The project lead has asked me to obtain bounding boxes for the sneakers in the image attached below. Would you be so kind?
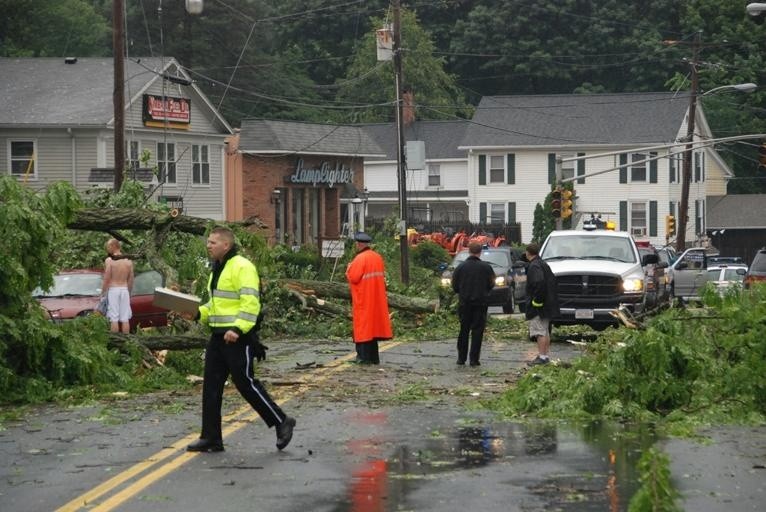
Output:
[188,437,224,452]
[528,357,549,366]
[276,417,296,449]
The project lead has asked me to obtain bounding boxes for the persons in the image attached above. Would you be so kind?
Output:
[452,243,496,364]
[187,226,296,453]
[525,243,559,367]
[101,237,134,336]
[345,233,392,364]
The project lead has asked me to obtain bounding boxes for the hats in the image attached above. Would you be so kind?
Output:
[355,235,371,243]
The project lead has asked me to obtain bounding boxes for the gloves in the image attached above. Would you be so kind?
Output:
[255,341,268,362]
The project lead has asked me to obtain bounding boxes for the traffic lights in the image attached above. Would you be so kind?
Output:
[551,187,563,219]
[665,215,675,238]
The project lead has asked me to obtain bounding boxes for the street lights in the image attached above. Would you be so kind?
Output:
[746,2,766,18]
[675,82,757,257]
[109,0,205,196]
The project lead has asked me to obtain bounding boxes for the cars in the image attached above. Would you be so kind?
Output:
[29,268,170,333]
[440,245,529,313]
[537,220,766,330]
